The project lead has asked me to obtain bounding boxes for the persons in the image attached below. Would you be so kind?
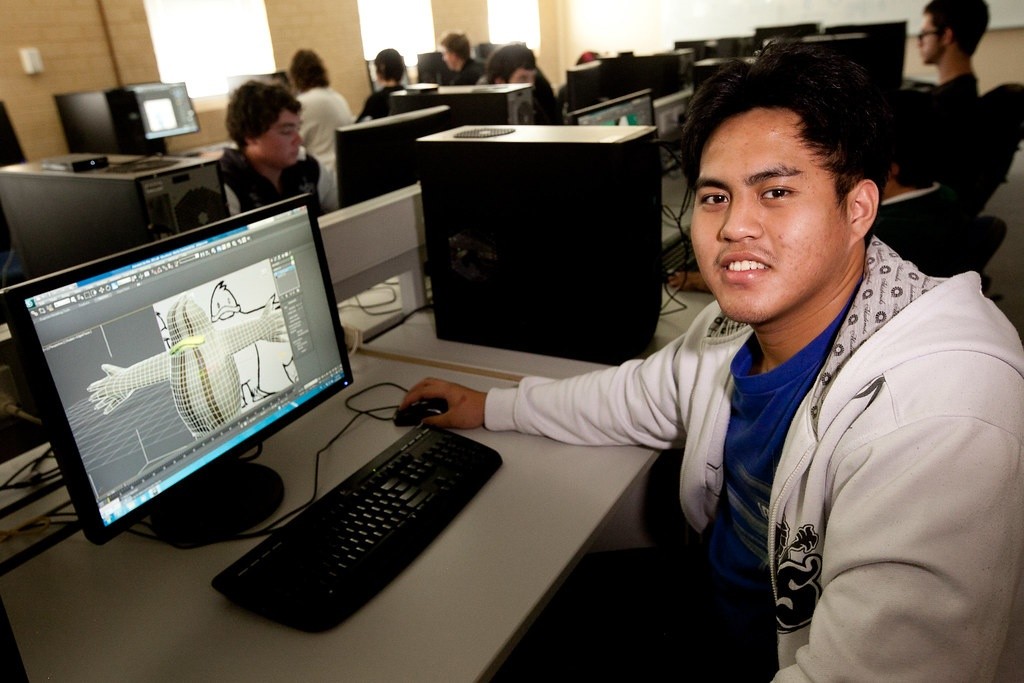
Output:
[915,0,991,138]
[289,49,357,208]
[399,34,1024,682]
[355,49,407,127]
[424,34,609,120]
[217,80,339,218]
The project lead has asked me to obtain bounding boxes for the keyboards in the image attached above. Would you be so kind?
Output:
[660,222,700,285]
[213,423,503,634]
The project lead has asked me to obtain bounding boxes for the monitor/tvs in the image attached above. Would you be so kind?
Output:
[366,60,411,94]
[565,89,655,127]
[244,73,291,93]
[565,60,603,111]
[334,106,449,209]
[125,82,200,140]
[0,190,355,551]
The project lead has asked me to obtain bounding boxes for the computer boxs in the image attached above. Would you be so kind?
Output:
[0,20,907,367]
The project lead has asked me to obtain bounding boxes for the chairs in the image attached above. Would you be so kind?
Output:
[916,83,1024,283]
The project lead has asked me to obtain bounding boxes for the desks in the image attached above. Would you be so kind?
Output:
[0,169,717,683]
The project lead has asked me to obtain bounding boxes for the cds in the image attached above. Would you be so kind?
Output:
[455,127,516,139]
[104,159,179,173]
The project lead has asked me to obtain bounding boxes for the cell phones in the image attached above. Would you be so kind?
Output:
[43,153,109,172]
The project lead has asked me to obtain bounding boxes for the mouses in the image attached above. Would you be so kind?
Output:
[392,398,448,428]
